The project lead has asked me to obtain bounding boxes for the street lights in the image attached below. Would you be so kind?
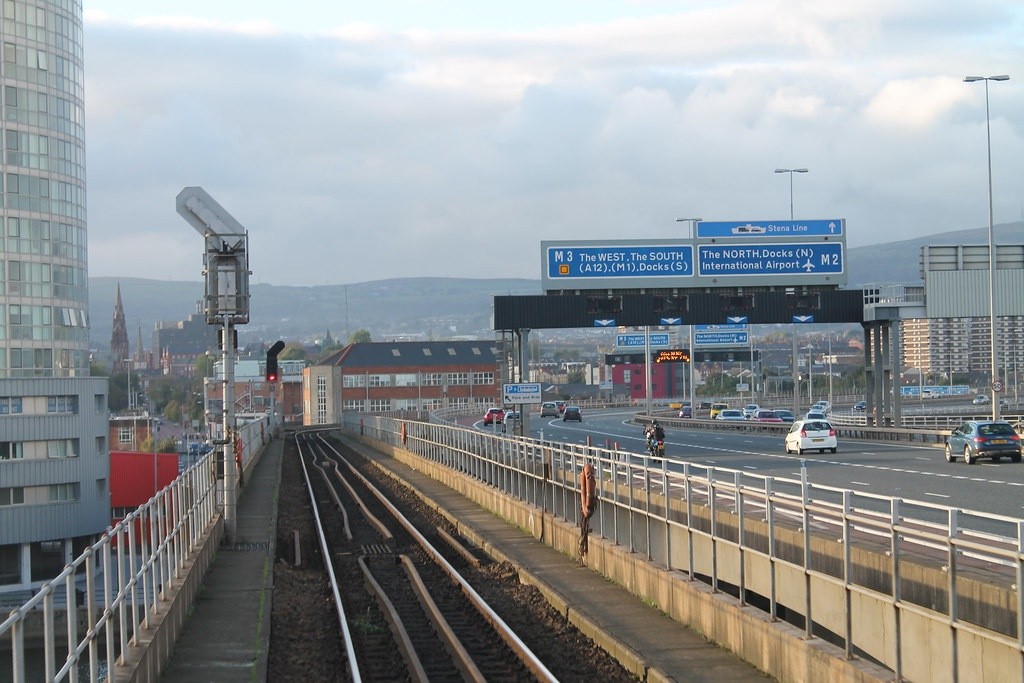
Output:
[945,338,952,396]
[827,330,835,406]
[774,167,809,220]
[962,75,1012,422]
[675,217,703,238]
[124,358,134,440]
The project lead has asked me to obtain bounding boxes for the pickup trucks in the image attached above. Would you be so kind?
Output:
[922,390,941,399]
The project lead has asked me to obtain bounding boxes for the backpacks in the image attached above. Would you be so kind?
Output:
[652,427,665,441]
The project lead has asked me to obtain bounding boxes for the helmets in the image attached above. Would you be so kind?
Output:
[654,424,659,428]
[651,418,657,423]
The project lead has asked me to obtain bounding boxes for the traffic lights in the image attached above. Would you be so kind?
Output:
[266,340,286,384]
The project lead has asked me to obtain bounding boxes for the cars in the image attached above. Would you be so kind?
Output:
[553,400,570,414]
[563,405,583,422]
[943,421,1022,465]
[153,417,214,476]
[784,419,838,455]
[1011,422,1024,435]
[500,410,521,433]
[972,395,990,405]
[539,402,560,419]
[853,401,867,412]
[668,400,831,432]
[483,407,505,427]
[1000,400,1008,410]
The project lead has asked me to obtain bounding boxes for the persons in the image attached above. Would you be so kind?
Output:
[643,418,665,456]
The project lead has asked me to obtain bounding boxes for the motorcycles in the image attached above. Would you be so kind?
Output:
[640,425,665,463]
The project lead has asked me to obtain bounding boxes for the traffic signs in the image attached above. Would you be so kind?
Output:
[502,383,542,405]
[697,218,844,238]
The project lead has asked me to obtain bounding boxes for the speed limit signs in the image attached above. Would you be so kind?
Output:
[991,381,1003,392]
